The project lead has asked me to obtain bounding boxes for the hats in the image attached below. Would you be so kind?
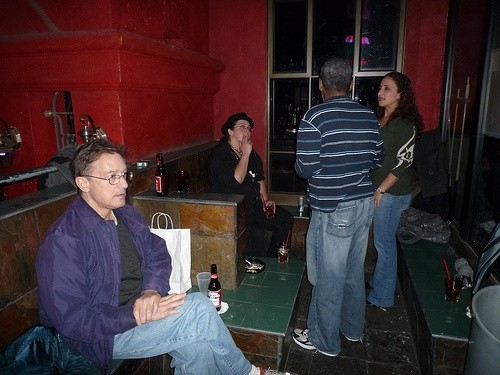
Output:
[221,112,254,137]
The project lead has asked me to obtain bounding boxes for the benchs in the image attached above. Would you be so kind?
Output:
[275,204,313,260]
[186,254,306,375]
[155,139,220,192]
[397,236,484,375]
[0,183,164,375]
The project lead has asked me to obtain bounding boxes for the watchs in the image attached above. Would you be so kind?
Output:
[377,188,385,194]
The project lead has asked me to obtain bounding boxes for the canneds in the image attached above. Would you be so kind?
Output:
[298,205,304,213]
[298,196,304,205]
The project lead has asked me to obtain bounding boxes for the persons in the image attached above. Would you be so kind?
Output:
[35,138,297,375]
[203,113,294,257]
[366,71,425,307]
[293,55,384,357]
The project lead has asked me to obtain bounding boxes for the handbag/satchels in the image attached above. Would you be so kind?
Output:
[148,212,192,295]
[396,206,451,244]
[0,326,102,375]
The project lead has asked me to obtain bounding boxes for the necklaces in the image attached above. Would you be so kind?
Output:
[231,145,240,154]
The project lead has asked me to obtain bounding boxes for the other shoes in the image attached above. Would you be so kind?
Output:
[260,365,297,375]
[366,300,372,305]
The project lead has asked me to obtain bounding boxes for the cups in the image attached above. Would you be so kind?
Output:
[446,274,461,299]
[196,271,210,296]
[278,241,289,264]
[175,169,189,194]
[264,202,274,218]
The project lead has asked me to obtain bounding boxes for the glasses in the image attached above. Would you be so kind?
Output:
[234,125,250,130]
[81,172,133,185]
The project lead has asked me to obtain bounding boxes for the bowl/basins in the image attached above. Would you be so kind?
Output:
[245,259,266,273]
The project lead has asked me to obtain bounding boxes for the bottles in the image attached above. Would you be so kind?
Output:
[206,263,222,311]
[155,153,169,197]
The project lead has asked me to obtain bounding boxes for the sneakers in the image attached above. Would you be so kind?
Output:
[292,328,341,356]
[345,335,359,341]
